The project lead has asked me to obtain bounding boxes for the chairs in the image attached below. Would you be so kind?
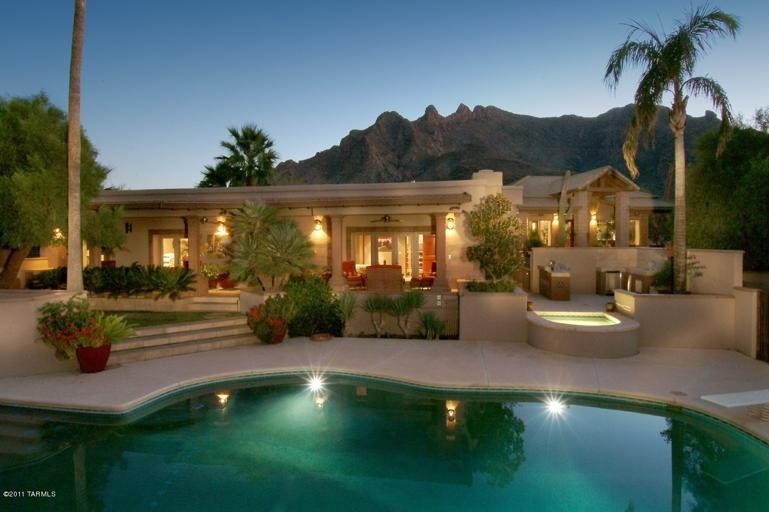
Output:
[343,260,438,290]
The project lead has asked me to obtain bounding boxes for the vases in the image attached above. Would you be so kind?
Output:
[77,343,111,373]
[655,286,692,294]
[259,321,293,344]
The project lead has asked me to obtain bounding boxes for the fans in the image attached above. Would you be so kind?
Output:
[369,215,406,225]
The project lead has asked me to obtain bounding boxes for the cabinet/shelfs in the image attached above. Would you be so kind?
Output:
[539,265,570,301]
[595,266,657,297]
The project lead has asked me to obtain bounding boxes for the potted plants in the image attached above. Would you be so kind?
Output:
[458,194,529,344]
[290,276,344,342]
[203,263,239,289]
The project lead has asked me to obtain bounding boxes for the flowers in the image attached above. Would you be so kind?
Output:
[647,240,676,285]
[32,296,141,358]
[245,294,296,331]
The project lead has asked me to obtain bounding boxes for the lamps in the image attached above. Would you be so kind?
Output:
[313,215,324,230]
[216,219,225,234]
[447,213,456,231]
